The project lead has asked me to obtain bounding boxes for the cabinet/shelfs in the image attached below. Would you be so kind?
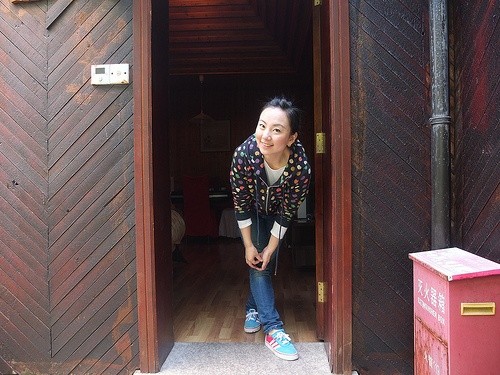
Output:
[287,220,315,272]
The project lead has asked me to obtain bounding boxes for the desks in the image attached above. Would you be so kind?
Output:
[173,194,184,199]
[209,188,230,197]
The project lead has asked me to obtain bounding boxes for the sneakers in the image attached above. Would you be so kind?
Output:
[244,309,261,333]
[265,329,298,361]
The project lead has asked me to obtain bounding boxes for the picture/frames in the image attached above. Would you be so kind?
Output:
[199,118,232,152]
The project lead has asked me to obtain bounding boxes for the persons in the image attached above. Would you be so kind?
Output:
[229,97,311,361]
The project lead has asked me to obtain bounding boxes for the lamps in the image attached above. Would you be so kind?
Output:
[188,75,216,125]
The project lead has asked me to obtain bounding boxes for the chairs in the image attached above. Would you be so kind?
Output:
[181,176,219,241]
